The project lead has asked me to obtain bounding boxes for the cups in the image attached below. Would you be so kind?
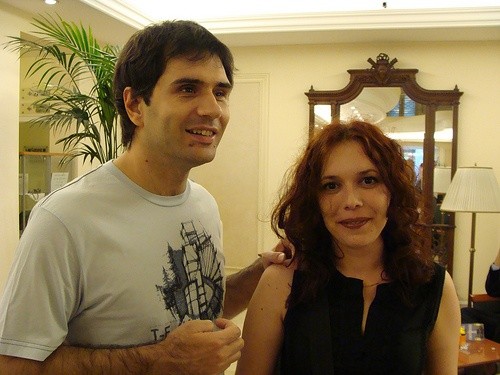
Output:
[460,323,485,358]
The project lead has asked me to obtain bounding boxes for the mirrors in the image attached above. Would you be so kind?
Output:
[303,57,464,282]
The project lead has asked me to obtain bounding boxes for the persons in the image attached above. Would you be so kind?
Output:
[235,120,461,375]
[469,249,500,344]
[0,19,296,375]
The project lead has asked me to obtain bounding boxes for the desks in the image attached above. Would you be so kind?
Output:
[457,328,500,375]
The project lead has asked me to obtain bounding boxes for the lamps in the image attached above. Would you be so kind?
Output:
[439,163,500,309]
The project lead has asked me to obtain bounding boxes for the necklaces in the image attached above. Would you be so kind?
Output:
[363,278,385,288]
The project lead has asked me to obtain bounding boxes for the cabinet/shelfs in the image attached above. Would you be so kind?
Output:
[18,150,76,238]
